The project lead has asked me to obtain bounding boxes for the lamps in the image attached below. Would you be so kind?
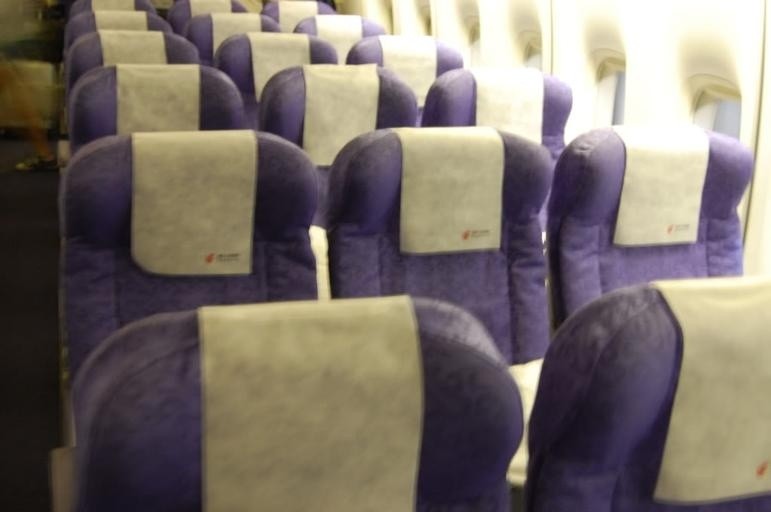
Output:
[65,28,201,100]
[56,130,316,388]
[419,67,575,234]
[61,293,525,512]
[182,12,282,67]
[258,64,418,234]
[293,14,388,65]
[546,120,755,328]
[214,29,339,130]
[526,274,771,512]
[324,125,554,511]
[260,1,339,33]
[344,33,464,126]
[67,0,159,21]
[63,9,176,53]
[65,62,246,159]
[166,0,251,36]
